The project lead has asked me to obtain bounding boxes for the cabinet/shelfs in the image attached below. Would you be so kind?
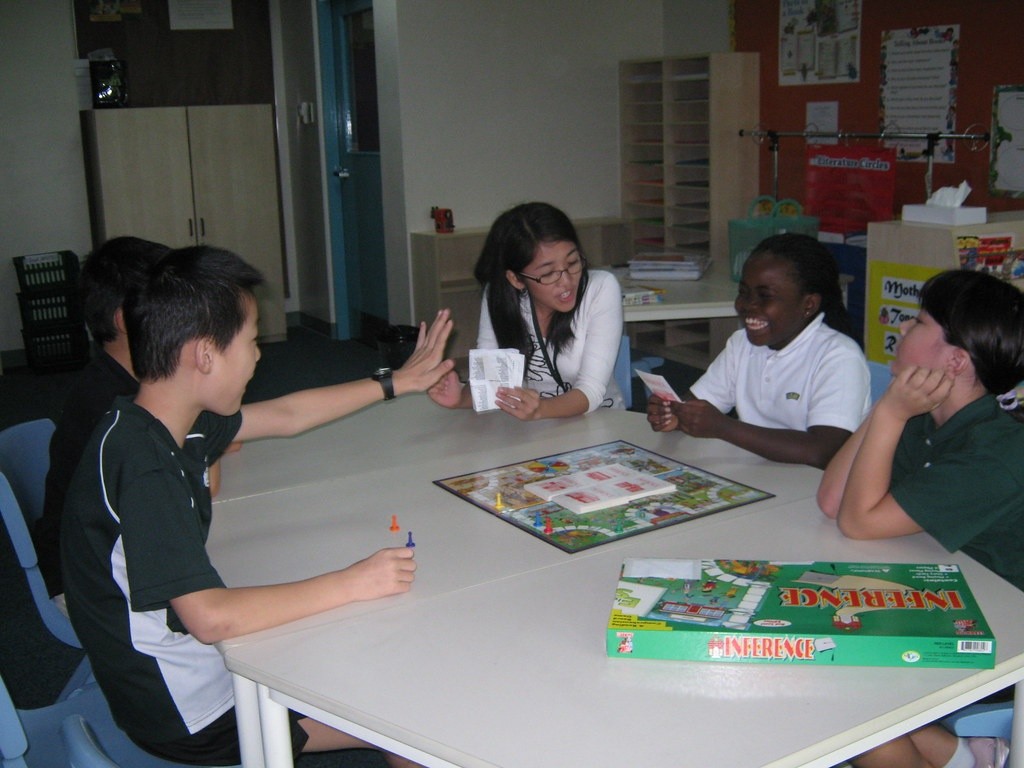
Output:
[618,51,761,370]
[80,103,287,344]
[410,217,635,363]
[865,210,1024,368]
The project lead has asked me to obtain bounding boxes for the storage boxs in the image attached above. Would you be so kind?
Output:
[13,250,90,372]
[605,556,996,669]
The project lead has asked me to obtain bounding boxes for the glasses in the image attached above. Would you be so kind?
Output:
[518,256,587,285]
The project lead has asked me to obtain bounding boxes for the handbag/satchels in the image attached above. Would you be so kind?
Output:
[727,196,820,282]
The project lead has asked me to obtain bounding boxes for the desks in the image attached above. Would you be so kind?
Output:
[208,383,1024,768]
[595,258,854,322]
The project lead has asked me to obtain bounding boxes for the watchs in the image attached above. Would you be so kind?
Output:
[372,367,396,400]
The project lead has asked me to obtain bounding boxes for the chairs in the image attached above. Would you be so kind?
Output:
[0,675,202,768]
[0,417,97,707]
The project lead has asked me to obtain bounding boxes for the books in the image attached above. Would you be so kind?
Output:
[620,251,713,305]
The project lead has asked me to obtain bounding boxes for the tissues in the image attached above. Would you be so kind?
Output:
[901,179,987,225]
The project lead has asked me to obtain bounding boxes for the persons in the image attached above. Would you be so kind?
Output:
[816,269,1024,768]
[59,246,455,768]
[425,202,626,421]
[39,236,174,620]
[646,233,873,470]
